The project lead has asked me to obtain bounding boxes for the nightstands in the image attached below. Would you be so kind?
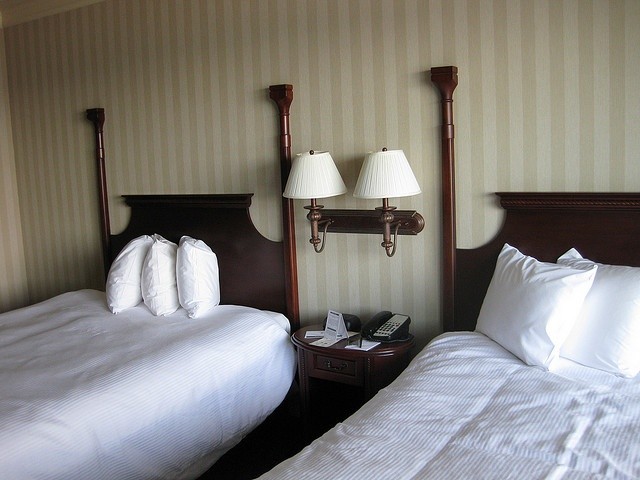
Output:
[291,325,416,400]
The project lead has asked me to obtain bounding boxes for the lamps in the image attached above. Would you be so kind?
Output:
[282,150,347,254]
[353,146,421,257]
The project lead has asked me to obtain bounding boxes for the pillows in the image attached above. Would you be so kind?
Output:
[141,239,179,316]
[554,245,640,379]
[105,235,153,313]
[475,242,599,371]
[176,235,220,319]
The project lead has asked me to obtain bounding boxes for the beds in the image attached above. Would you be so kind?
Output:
[257,67,640,479]
[0,83,298,479]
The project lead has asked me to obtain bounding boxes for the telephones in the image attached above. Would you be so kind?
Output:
[361,311,411,342]
